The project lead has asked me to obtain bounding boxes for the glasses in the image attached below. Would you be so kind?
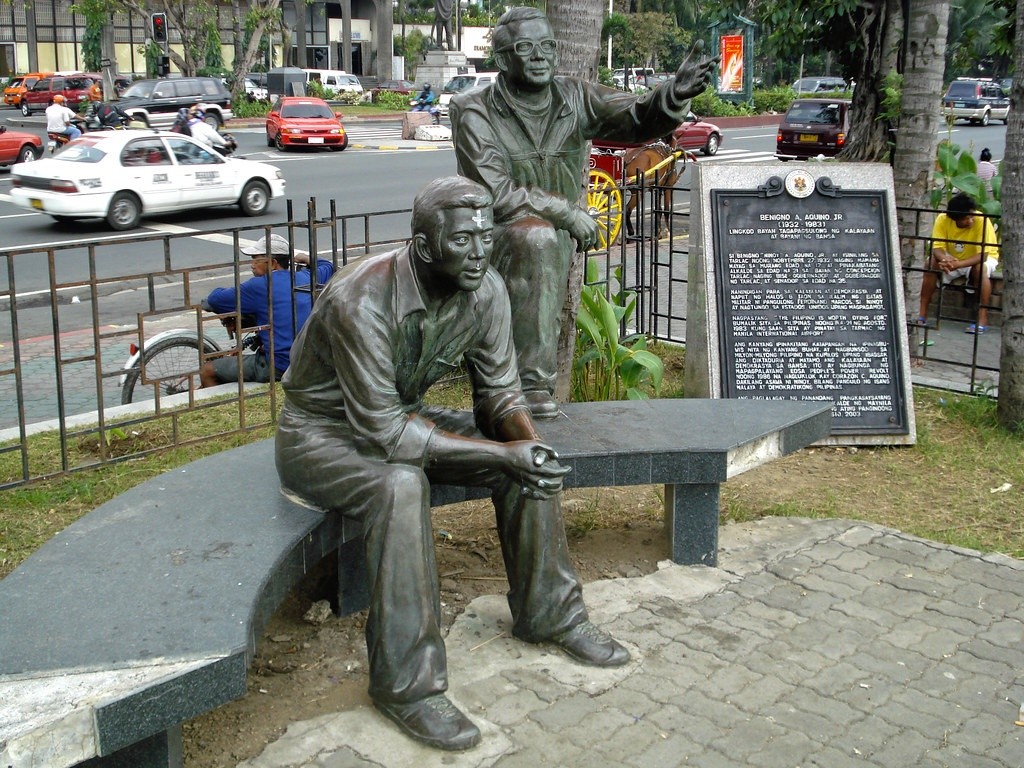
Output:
[252,259,269,265]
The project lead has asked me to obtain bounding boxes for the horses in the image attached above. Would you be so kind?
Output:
[623,132,684,237]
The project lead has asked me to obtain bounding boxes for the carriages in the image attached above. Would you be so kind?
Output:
[587,132,704,251]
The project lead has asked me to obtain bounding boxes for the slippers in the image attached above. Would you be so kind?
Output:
[964,324,989,335]
[912,318,931,326]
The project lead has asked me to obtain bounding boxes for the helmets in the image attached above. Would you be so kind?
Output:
[424,83,431,91]
[53,95,67,106]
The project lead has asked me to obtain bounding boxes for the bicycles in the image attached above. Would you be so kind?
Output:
[118,257,309,407]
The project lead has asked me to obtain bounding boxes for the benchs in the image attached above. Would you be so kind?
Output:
[0,400,834,768]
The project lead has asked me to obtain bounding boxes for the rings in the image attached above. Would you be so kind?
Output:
[941,259,945,262]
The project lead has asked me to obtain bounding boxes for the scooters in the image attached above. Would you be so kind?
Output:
[408,97,442,125]
[47,115,85,156]
[213,131,249,160]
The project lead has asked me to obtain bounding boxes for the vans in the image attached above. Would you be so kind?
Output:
[105,76,234,129]
[438,72,500,117]
[791,76,846,93]
[3,71,144,117]
[219,66,364,104]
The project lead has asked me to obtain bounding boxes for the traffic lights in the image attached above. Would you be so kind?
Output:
[151,13,168,43]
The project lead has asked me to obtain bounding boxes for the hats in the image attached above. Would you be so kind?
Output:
[241,234,289,254]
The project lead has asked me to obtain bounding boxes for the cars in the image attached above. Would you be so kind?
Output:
[8,129,286,231]
[674,111,724,157]
[266,96,348,153]
[773,97,853,163]
[367,79,420,97]
[0,124,44,168]
[608,68,675,94]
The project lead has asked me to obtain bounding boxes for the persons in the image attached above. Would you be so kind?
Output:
[88,79,102,100]
[277,174,631,751]
[45,95,88,141]
[917,192,999,334]
[93,101,134,130]
[114,82,125,92]
[409,82,434,112]
[171,106,231,157]
[199,233,334,388]
[449,6,723,419]
[977,148,999,196]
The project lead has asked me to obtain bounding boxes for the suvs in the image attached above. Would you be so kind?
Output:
[942,77,1012,127]
